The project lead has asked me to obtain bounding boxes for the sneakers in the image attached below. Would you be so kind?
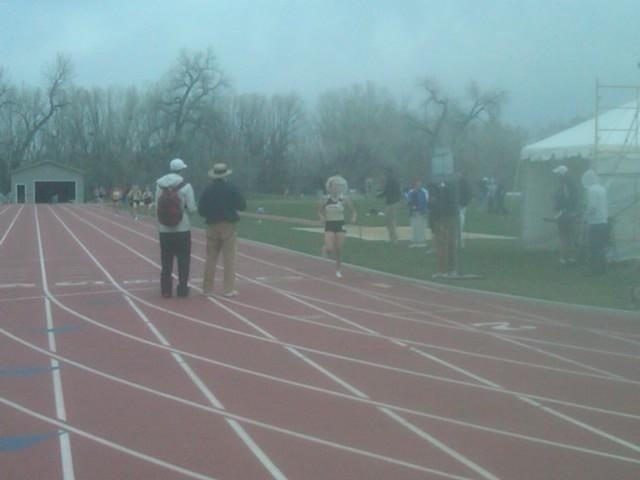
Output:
[432,272,456,278]
[203,291,239,297]
[409,244,428,248]
[336,271,342,278]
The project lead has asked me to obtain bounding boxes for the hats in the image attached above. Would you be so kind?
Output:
[552,165,567,175]
[207,163,230,178]
[170,158,187,173]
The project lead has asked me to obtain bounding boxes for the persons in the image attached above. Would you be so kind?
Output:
[197,163,247,299]
[578,167,611,276]
[316,174,357,280]
[550,164,585,267]
[153,156,199,300]
[455,166,474,249]
[476,175,508,216]
[373,164,404,244]
[91,179,154,224]
[403,176,431,249]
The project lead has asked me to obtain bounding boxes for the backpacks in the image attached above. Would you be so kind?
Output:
[157,183,186,226]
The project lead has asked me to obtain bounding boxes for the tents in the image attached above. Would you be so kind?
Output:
[516,97,639,263]
